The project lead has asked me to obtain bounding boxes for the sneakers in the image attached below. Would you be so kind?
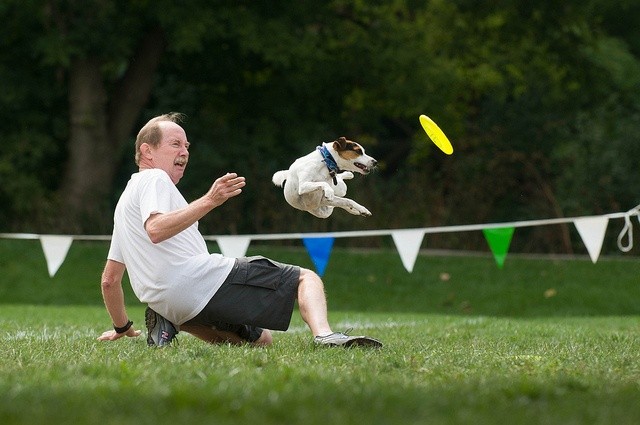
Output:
[144,307,179,348]
[312,327,386,349]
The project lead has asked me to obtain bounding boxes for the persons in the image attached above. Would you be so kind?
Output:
[97,113,382,347]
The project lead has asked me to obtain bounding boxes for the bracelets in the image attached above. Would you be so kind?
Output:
[112,321,133,333]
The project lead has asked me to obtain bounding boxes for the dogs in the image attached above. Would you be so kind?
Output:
[271,136,378,219]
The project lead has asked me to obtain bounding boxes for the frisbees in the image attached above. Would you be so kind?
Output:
[419,114,454,155]
